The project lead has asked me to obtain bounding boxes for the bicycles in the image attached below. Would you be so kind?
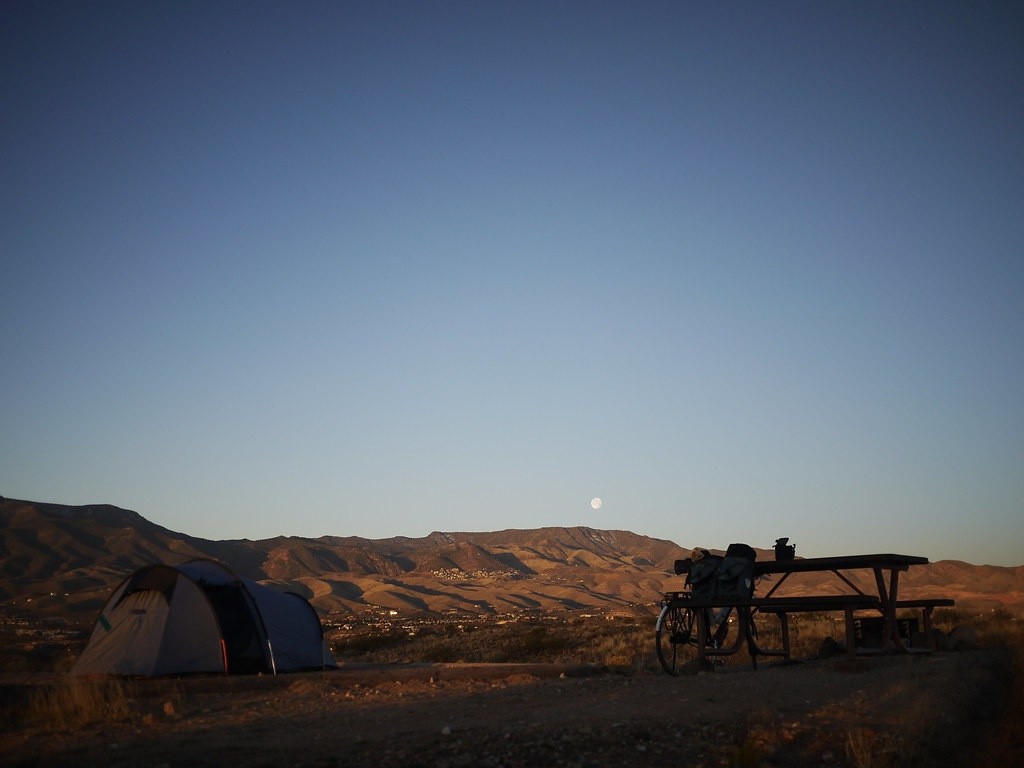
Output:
[655,552,773,676]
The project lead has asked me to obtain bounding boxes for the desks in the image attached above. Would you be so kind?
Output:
[703,553,932,659]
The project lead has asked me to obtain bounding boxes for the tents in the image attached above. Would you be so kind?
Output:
[71,557,338,675]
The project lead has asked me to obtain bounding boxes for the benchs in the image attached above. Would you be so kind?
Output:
[666,594,955,669]
